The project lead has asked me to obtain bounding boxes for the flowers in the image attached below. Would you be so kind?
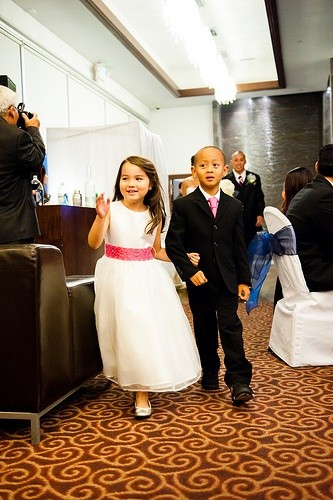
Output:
[246,173,256,186]
[220,179,235,197]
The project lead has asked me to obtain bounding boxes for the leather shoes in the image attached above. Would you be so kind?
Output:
[202,371,219,390]
[230,384,253,403]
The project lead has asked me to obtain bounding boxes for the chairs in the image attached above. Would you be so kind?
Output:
[244,205,333,367]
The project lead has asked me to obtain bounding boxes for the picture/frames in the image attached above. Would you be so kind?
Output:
[168,174,192,215]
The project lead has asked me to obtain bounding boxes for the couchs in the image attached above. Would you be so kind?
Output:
[0,243,103,445]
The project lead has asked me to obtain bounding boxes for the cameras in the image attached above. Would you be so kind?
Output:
[17,111,33,129]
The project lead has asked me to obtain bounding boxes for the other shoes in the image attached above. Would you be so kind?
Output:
[135,399,151,416]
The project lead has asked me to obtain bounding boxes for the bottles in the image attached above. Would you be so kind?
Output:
[58,181,68,205]
[85,164,96,208]
[72,190,81,207]
[31,175,44,206]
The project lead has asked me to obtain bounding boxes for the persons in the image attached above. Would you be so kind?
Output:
[165,146,254,404]
[285,142,333,292]
[88,155,204,417]
[273,167,313,310]
[176,156,200,200]
[221,151,264,302]
[0,84,47,246]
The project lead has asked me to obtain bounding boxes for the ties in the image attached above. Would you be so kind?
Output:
[207,197,219,218]
[238,176,243,187]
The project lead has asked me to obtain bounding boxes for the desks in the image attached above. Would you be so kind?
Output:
[34,206,105,277]
